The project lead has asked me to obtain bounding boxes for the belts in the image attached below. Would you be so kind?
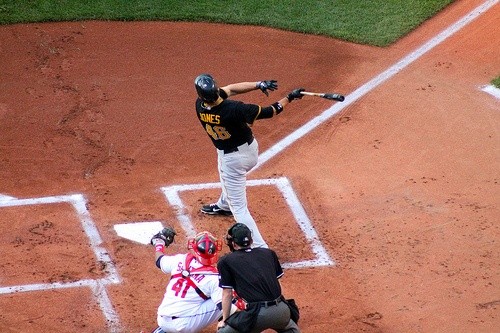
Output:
[224,134,255,154]
[253,296,285,306]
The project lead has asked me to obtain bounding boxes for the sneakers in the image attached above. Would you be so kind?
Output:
[200,203,233,216]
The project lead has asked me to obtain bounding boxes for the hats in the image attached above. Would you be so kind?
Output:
[231,223,253,246]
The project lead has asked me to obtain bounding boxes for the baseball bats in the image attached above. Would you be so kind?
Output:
[295,89,346,104]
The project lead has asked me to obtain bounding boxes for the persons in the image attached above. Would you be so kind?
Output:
[152,227,249,333]
[217,223,302,333]
[194,74,304,251]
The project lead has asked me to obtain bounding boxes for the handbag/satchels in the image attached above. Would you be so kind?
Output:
[281,295,300,323]
[224,302,261,333]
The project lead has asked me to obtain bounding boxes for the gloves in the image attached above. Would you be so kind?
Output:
[256,80,278,96]
[287,88,305,103]
[152,237,165,247]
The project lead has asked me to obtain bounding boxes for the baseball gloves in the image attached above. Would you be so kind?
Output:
[150,226,176,247]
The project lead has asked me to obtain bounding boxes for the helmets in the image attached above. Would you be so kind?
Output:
[196,232,217,255]
[194,73,218,103]
